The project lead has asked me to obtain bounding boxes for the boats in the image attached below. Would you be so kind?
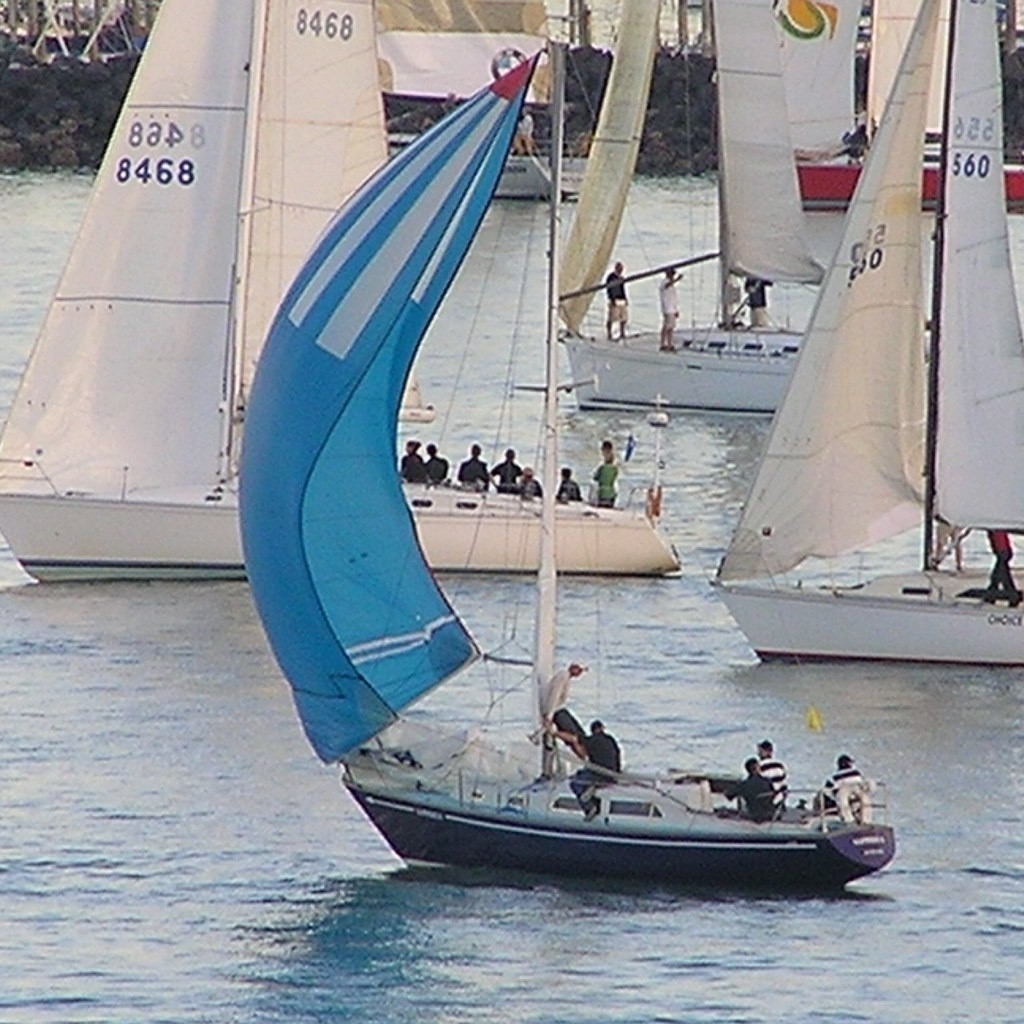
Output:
[388,132,579,201]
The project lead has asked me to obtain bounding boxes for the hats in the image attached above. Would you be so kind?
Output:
[758,740,774,750]
[590,720,605,731]
[570,663,588,671]
[838,754,853,765]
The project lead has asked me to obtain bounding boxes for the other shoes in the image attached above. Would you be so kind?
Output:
[579,784,595,802]
[621,336,628,341]
[659,345,668,351]
[583,805,598,821]
[607,336,616,344]
[669,343,676,350]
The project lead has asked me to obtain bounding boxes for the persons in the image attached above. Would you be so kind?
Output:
[981,529,1020,607]
[490,449,523,495]
[935,521,964,571]
[423,443,449,484]
[756,740,788,809]
[520,467,541,500]
[514,106,535,156]
[726,757,776,823]
[658,267,683,350]
[555,718,622,819]
[401,441,426,483]
[745,277,773,327]
[606,261,629,342]
[556,468,582,504]
[813,753,867,819]
[842,106,878,166]
[589,441,622,509]
[541,658,587,746]
[457,444,489,492]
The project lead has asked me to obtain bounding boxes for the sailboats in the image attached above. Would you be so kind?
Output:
[229,35,895,892]
[708,1,1024,670]
[764,0,1024,214]
[1,0,684,583]
[561,1,916,421]
